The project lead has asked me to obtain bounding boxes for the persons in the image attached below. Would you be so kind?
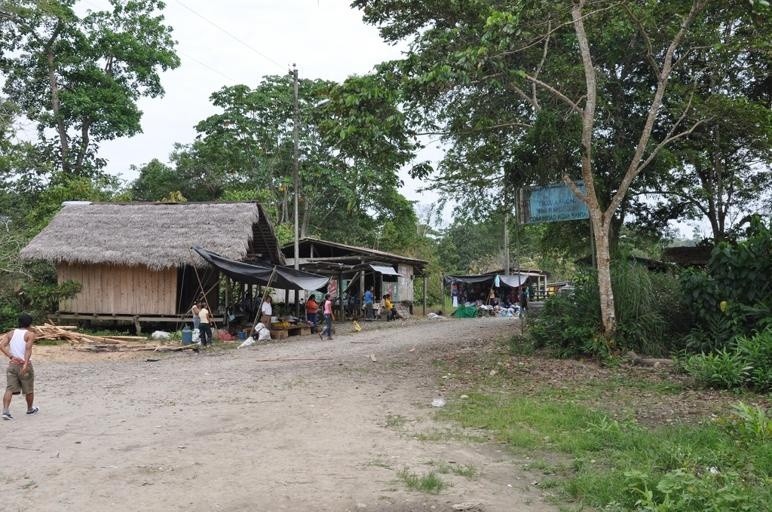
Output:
[519,288,529,318]
[192,301,212,346]
[381,294,408,321]
[306,294,320,334]
[362,286,375,319]
[0,314,38,420]
[318,294,335,341]
[261,296,273,329]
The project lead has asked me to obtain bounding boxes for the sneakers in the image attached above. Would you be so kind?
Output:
[26,408,38,415]
[319,333,334,341]
[1,411,12,420]
